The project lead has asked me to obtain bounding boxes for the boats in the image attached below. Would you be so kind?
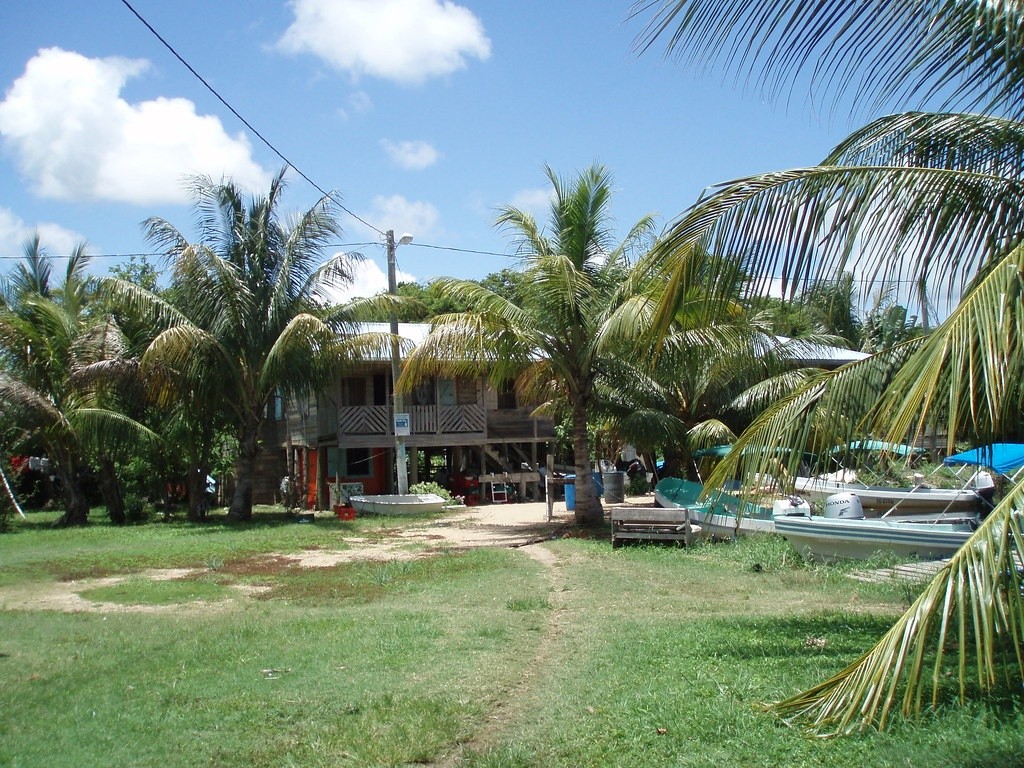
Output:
[348,493,446,515]
[794,472,996,524]
[746,466,858,497]
[771,493,1014,568]
[654,475,866,544]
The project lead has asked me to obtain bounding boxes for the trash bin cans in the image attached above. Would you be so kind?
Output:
[604,471,624,503]
[564,475,576,511]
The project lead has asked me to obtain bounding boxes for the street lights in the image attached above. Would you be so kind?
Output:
[382,229,415,492]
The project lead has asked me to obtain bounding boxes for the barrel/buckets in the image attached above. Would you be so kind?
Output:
[604,472,624,503]
[592,472,602,497]
[564,485,576,510]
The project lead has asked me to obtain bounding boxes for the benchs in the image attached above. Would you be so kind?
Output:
[611,507,701,547]
